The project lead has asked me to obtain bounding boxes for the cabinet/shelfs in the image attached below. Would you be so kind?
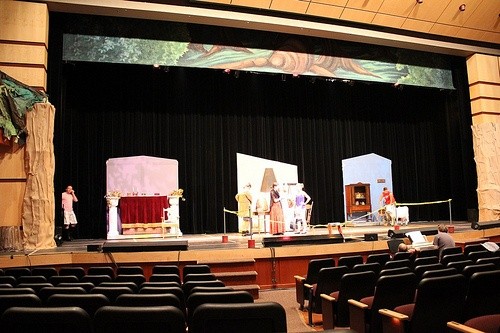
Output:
[346,182,372,222]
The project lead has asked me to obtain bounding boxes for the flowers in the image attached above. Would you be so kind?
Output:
[104,191,121,208]
[171,188,185,197]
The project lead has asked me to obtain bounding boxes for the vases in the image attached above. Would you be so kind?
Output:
[106,197,119,207]
[168,196,180,206]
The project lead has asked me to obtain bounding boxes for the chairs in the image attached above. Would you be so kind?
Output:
[161,207,179,239]
[0,265,287,333]
[294,240,500,333]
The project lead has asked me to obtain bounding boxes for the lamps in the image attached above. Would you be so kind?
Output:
[459,4,466,11]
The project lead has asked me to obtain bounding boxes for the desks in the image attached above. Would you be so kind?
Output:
[392,206,409,226]
[120,196,168,234]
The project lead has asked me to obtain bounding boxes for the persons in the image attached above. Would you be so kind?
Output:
[62,185,78,242]
[398,243,407,252]
[378,187,396,226]
[436,224,455,263]
[270,183,286,235]
[294,183,311,234]
[241,182,266,213]
